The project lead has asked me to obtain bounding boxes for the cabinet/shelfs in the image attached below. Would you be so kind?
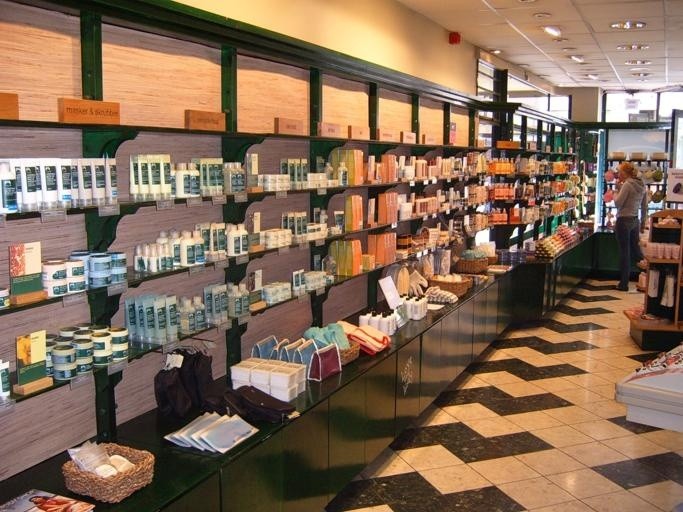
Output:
[0,1,683,512]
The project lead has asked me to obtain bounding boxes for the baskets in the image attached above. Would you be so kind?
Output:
[335,340,360,365]
[63,443,154,502]
[429,255,488,297]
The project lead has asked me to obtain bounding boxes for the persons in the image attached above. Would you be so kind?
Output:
[612,162,648,291]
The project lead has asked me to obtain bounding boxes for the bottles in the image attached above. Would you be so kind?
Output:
[176,295,207,333]
[172,233,180,264]
[133,242,172,272]
[489,157,515,174]
[171,162,201,197]
[227,282,250,317]
[156,231,168,243]
[399,293,429,320]
[489,183,514,200]
[193,231,205,264]
[181,232,195,266]
[321,254,337,277]
[359,307,396,336]
[223,162,245,195]
[320,209,328,224]
[227,226,241,256]
[325,161,348,186]
[490,208,508,222]
[238,224,249,254]
